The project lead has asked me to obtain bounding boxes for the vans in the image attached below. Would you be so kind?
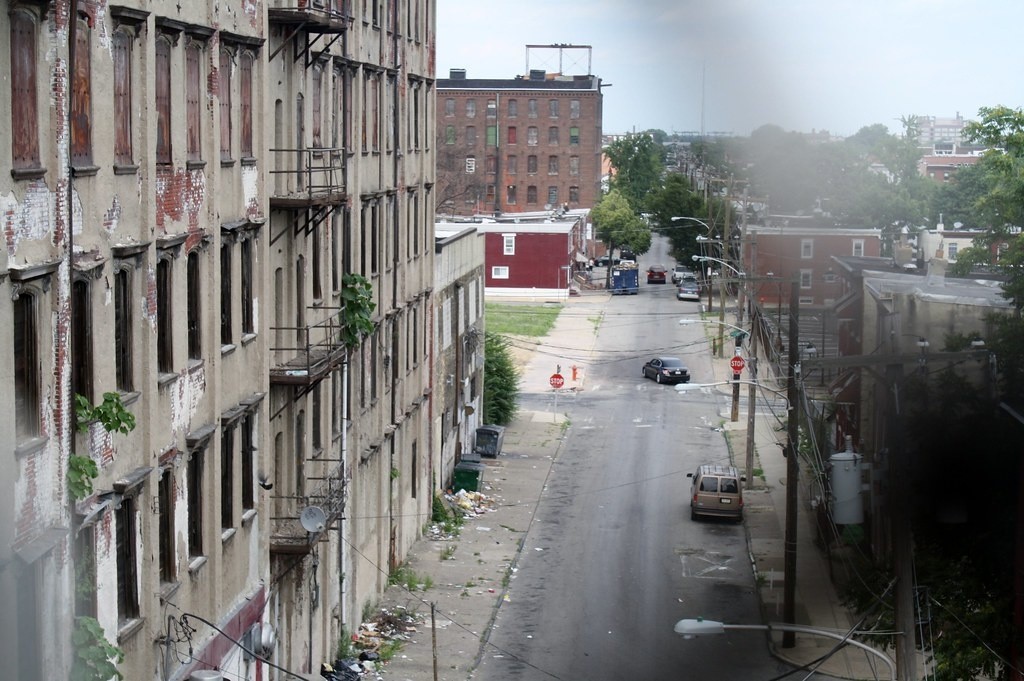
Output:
[620,245,636,262]
[686,464,747,521]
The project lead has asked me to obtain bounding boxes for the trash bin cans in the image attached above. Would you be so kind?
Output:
[453,453,481,492]
[475,425,505,459]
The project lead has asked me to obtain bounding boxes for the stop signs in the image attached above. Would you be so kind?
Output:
[550,373,565,389]
[730,356,745,371]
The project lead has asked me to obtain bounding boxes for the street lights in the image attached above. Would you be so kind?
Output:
[692,255,745,422]
[674,380,798,647]
[674,618,920,681]
[679,319,758,489]
[695,235,725,358]
[671,217,713,313]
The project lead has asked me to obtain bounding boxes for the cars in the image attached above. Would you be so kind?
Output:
[642,356,691,384]
[619,260,634,270]
[646,265,668,284]
[593,255,626,266]
[676,282,700,301]
[679,277,702,293]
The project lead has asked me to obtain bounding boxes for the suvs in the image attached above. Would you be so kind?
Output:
[672,263,693,283]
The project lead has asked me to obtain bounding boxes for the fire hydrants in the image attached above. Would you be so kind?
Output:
[572,365,577,380]
